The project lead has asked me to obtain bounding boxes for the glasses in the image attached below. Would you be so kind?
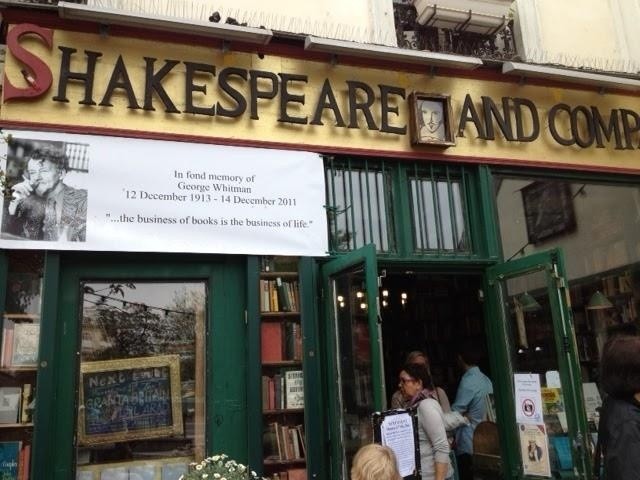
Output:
[399,379,415,383]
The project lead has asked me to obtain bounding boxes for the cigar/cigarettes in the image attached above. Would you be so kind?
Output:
[22,174,31,184]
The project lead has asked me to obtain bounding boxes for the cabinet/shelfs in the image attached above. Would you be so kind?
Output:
[260,272,307,464]
[0,313,40,428]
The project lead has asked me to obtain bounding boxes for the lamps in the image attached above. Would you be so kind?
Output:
[586,288,614,309]
[502,61,640,93]
[304,35,484,73]
[511,293,541,313]
[337,290,408,310]
[57,2,273,46]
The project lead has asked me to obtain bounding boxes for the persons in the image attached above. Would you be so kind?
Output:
[1,147,87,243]
[593,336,640,480]
[90,390,136,463]
[351,346,498,479]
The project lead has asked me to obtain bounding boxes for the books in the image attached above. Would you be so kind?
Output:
[0,317,41,480]
[541,381,605,471]
[258,277,308,480]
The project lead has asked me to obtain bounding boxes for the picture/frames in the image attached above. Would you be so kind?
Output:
[409,91,456,147]
